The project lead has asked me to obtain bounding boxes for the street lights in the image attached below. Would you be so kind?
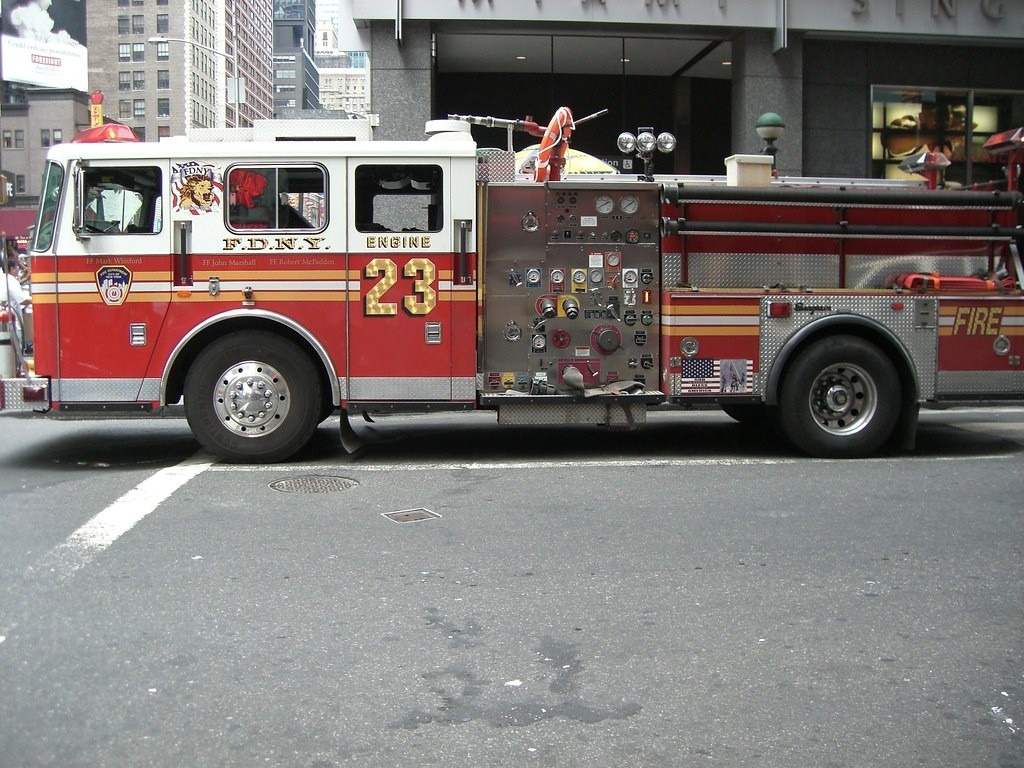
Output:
[147,0,239,127]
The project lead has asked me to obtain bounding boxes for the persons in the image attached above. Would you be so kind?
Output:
[229,167,289,229]
[0,236,33,378]
[11,0,79,46]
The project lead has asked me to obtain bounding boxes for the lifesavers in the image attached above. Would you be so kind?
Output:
[534,107,574,182]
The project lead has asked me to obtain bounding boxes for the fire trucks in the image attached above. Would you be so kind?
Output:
[0,90,1023,465]
